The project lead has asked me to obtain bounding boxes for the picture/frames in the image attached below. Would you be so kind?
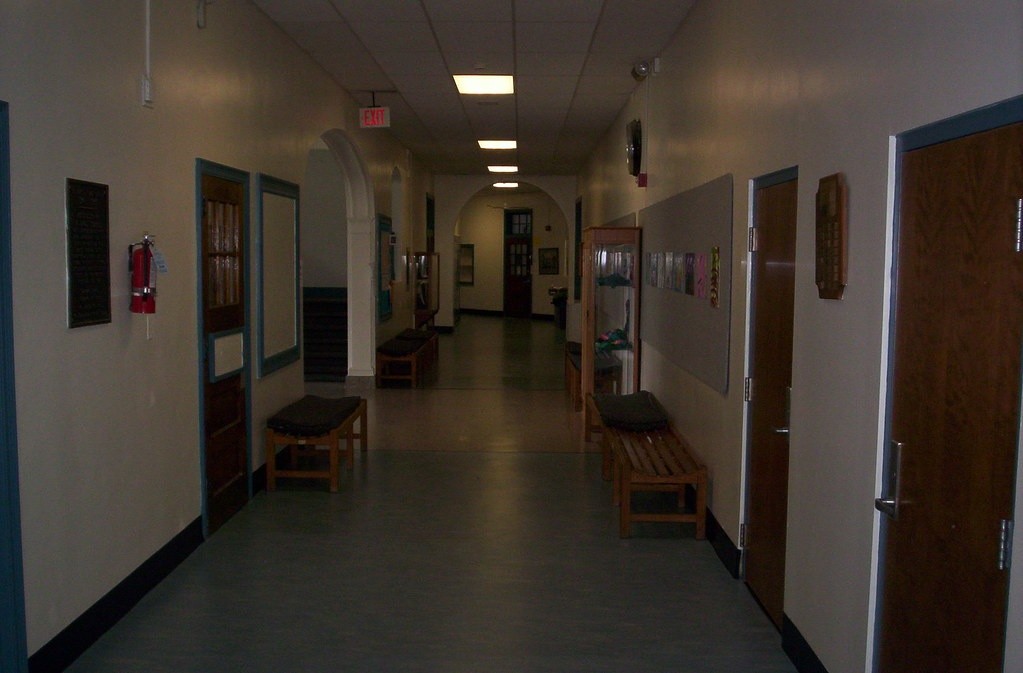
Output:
[538,247,560,274]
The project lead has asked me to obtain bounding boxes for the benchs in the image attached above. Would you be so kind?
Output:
[264,394,368,495]
[564,341,623,404]
[375,327,440,389]
[584,390,709,541]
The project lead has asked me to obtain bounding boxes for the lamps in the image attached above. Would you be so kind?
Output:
[631,57,660,82]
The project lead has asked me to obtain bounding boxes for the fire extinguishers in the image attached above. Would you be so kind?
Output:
[130,233,159,315]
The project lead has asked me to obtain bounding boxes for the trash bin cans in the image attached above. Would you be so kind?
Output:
[553,295,568,328]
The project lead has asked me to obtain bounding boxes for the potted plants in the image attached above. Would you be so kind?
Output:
[547,287,568,330]
